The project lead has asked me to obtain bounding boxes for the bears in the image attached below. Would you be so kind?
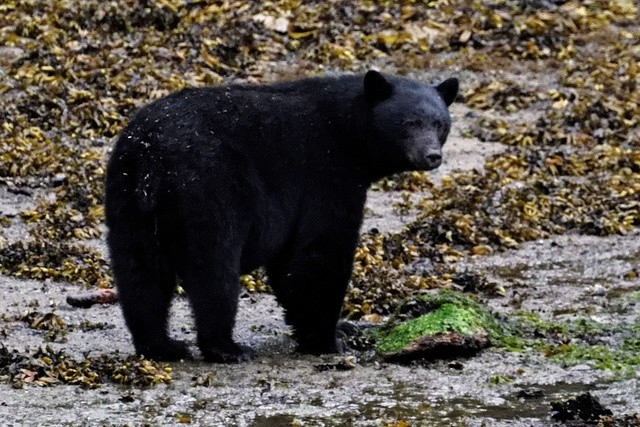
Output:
[104,69,459,365]
